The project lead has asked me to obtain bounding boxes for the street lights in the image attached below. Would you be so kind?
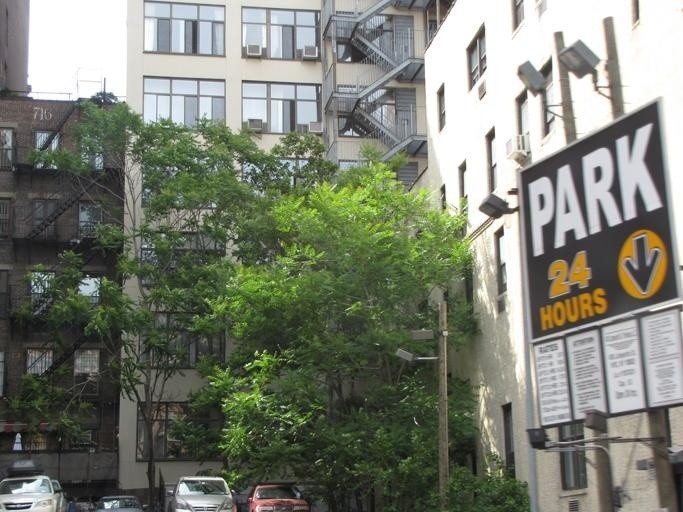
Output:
[395,301,449,511]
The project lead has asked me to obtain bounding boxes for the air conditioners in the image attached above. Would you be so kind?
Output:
[505,135,528,161]
[303,46,319,59]
[247,44,262,56]
[309,122,324,134]
[248,119,263,130]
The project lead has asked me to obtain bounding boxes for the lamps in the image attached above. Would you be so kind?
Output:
[517,40,615,118]
[477,195,518,219]
[526,409,662,452]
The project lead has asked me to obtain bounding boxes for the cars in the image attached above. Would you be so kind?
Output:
[0,474,70,512]
[247,482,310,512]
[69,495,150,512]
[167,476,238,512]
